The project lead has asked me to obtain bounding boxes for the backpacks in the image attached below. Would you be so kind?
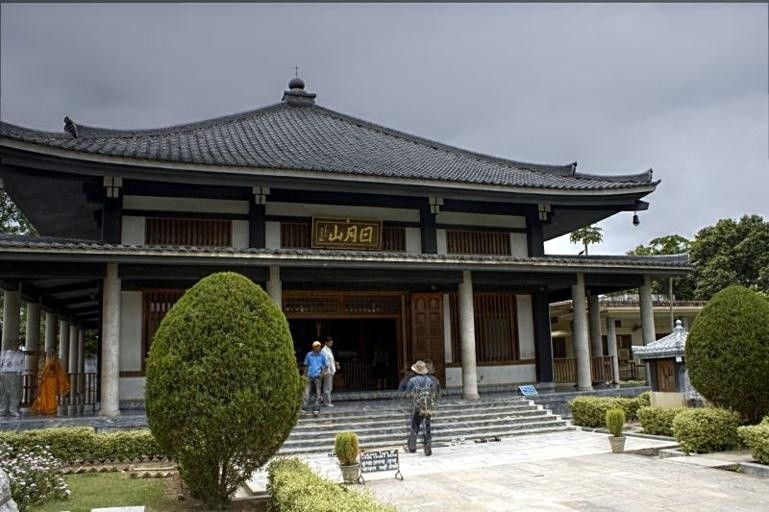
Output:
[411,377,436,417]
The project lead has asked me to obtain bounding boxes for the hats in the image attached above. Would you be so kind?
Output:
[312,340,322,347]
[410,360,429,375]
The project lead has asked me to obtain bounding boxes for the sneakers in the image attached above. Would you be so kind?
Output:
[321,403,335,407]
[300,409,321,416]
[404,445,432,456]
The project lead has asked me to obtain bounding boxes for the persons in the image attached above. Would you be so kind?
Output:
[321,338,336,408]
[0,338,26,417]
[402,360,436,455]
[31,350,71,415]
[299,340,329,416]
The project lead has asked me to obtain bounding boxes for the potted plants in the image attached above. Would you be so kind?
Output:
[606,410,627,454]
[335,432,362,486]
